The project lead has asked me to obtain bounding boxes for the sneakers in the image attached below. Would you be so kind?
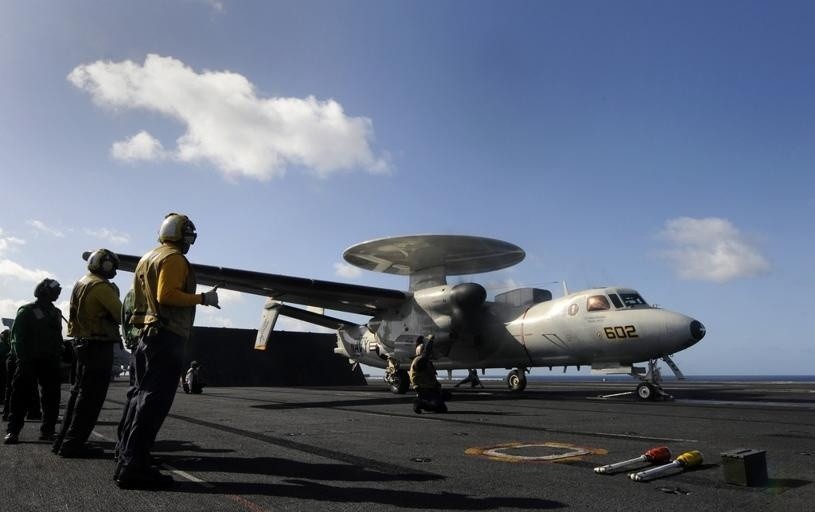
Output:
[113,467,174,491]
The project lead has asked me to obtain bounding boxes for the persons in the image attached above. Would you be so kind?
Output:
[182,360,209,394]
[104,210,222,493]
[1,279,63,443]
[51,248,123,460]
[410,333,448,414]
[451,368,481,388]
[0,325,45,422]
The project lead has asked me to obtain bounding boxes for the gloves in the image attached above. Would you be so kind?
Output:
[425,334,436,340]
[202,285,221,309]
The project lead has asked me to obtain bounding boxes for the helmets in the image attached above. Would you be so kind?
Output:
[87,248,119,277]
[34,278,61,302]
[158,212,196,243]
[415,342,424,356]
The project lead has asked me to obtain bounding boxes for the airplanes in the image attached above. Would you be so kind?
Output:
[82,233,706,400]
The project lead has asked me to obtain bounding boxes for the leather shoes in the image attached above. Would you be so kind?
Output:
[39,433,59,440]
[51,441,105,458]
[5,433,18,443]
[412,398,422,414]
